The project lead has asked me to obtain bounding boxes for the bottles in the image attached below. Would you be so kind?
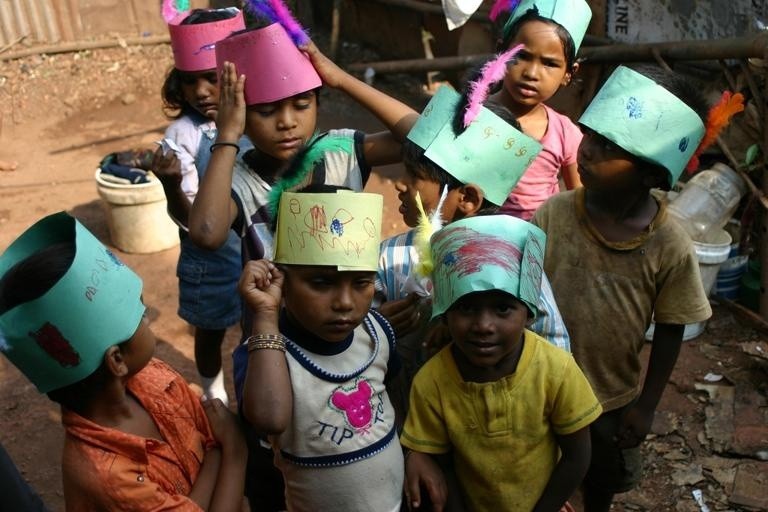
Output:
[666,162,746,242]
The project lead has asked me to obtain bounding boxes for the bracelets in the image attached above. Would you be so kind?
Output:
[209,142,241,154]
[247,334,288,353]
[204,440,219,450]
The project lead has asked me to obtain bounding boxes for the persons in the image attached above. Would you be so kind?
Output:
[398,214,603,512]
[151,6,256,408]
[531,62,713,512]
[376,80,572,355]
[1,212,249,512]
[474,0,594,223]
[233,186,408,511]
[187,22,422,264]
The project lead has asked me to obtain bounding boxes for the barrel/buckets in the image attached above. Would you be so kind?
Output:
[94,167,178,252]
[712,219,750,298]
[646,227,732,342]
